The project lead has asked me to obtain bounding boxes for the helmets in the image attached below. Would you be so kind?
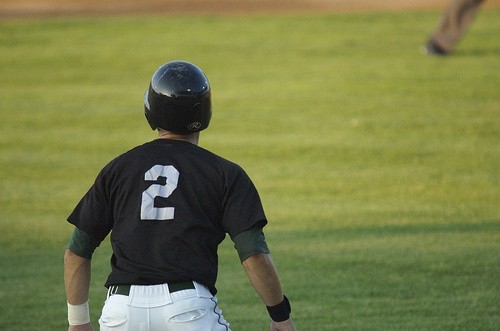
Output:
[144,60,213,132]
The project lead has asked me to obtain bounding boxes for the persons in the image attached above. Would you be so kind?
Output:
[64,60,295,331]
